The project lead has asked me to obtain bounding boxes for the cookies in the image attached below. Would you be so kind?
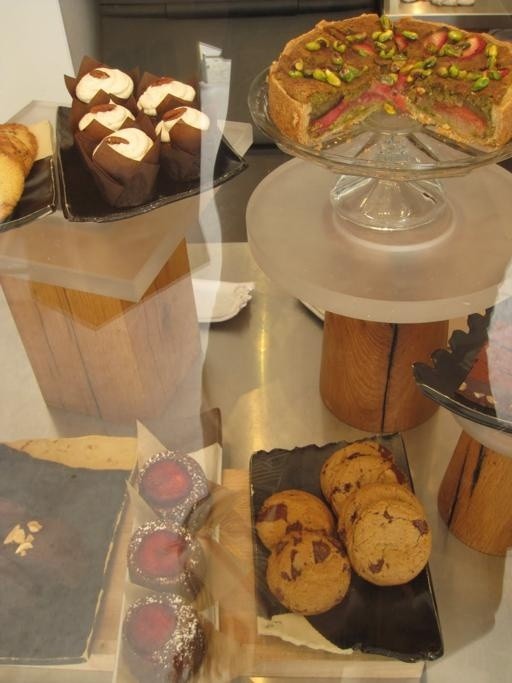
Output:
[255,441,433,616]
[0,122,38,224]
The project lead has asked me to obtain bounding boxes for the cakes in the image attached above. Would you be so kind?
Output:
[126,518,206,606]
[64,54,221,210]
[267,12,512,151]
[120,592,207,683]
[134,449,211,529]
